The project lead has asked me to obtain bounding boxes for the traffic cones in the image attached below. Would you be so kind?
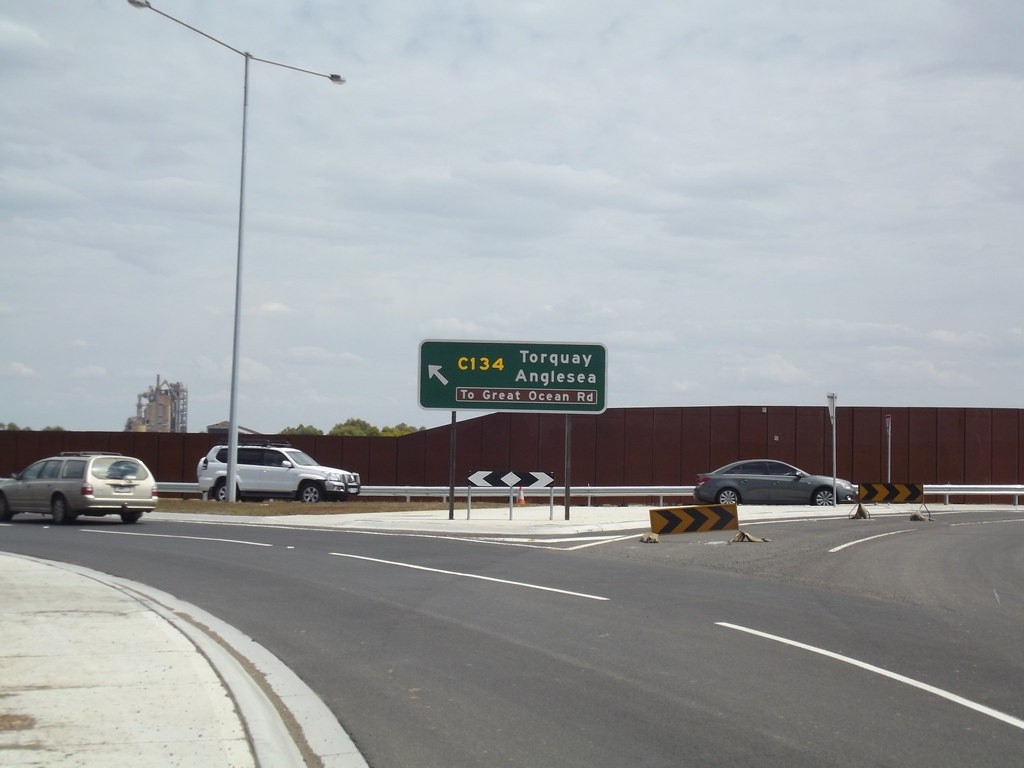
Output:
[516,486,526,506]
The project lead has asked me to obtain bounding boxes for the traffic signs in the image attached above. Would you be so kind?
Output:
[417,338,609,415]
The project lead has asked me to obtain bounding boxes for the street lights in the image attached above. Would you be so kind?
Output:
[124,0,349,505]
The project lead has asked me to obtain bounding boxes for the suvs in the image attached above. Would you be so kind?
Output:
[0,450,159,526]
[196,436,361,505]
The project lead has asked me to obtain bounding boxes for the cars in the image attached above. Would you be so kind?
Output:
[693,459,857,507]
